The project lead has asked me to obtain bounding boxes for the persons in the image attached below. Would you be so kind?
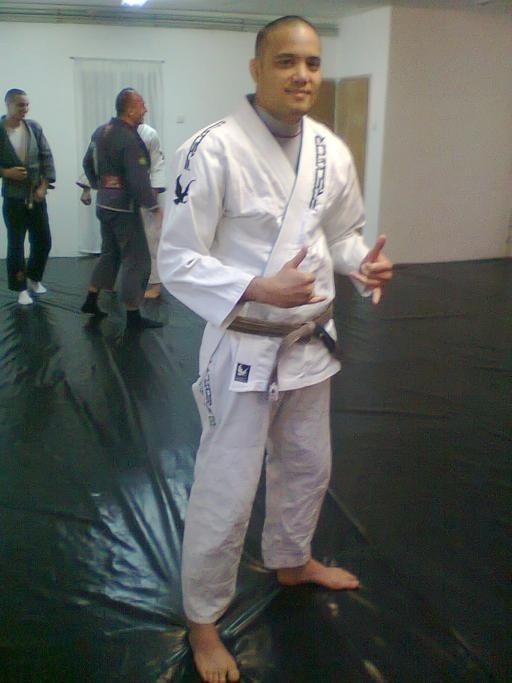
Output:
[1,87,57,307]
[155,17,395,683]
[77,88,168,331]
